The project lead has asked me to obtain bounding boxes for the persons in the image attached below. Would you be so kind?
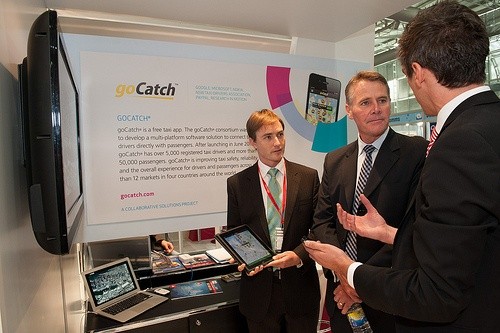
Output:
[312,69,431,333]
[304,0,500,333]
[225,108,322,333]
[150,234,174,255]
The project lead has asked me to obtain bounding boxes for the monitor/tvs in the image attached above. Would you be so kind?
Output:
[87,235,153,278]
[18,10,85,255]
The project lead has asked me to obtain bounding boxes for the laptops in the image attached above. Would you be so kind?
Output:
[81,257,168,322]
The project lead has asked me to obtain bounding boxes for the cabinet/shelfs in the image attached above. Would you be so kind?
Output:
[86,271,242,333]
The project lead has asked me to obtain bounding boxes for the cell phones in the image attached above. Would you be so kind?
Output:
[154,288,170,296]
[305,73,341,126]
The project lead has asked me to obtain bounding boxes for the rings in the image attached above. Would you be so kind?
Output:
[339,301,344,307]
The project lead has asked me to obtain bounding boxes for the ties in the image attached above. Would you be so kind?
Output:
[426,126,439,158]
[331,144,376,282]
[266,169,282,272]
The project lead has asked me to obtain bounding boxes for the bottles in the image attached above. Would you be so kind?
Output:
[347,302,373,333]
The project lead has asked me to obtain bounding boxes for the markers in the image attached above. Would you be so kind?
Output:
[208,279,216,293]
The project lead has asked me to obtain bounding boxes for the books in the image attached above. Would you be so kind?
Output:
[151,247,233,275]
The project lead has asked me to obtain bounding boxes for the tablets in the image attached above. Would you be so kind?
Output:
[214,224,276,274]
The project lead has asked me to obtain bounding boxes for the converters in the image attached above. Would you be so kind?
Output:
[179,254,193,264]
